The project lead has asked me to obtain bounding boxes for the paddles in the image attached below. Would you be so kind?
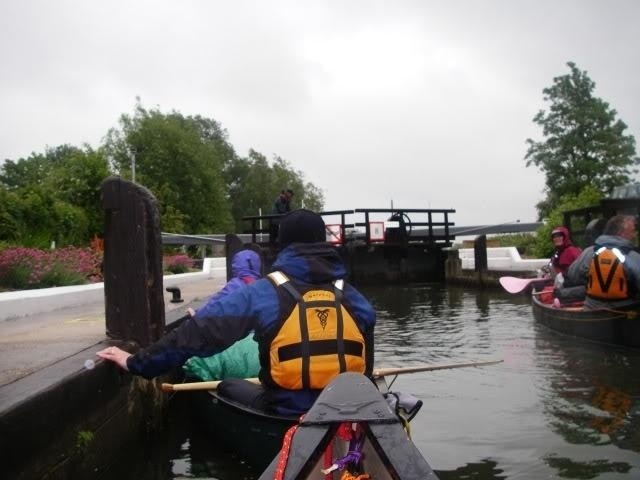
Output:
[161,359,504,393]
[499,276,545,294]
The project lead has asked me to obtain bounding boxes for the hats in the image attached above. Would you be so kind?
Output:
[278,209,328,247]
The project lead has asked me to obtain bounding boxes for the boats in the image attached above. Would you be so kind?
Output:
[531,286,640,341]
[201,376,390,446]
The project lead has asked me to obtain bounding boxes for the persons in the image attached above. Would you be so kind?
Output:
[270,188,295,245]
[95,210,377,416]
[539,215,640,307]
